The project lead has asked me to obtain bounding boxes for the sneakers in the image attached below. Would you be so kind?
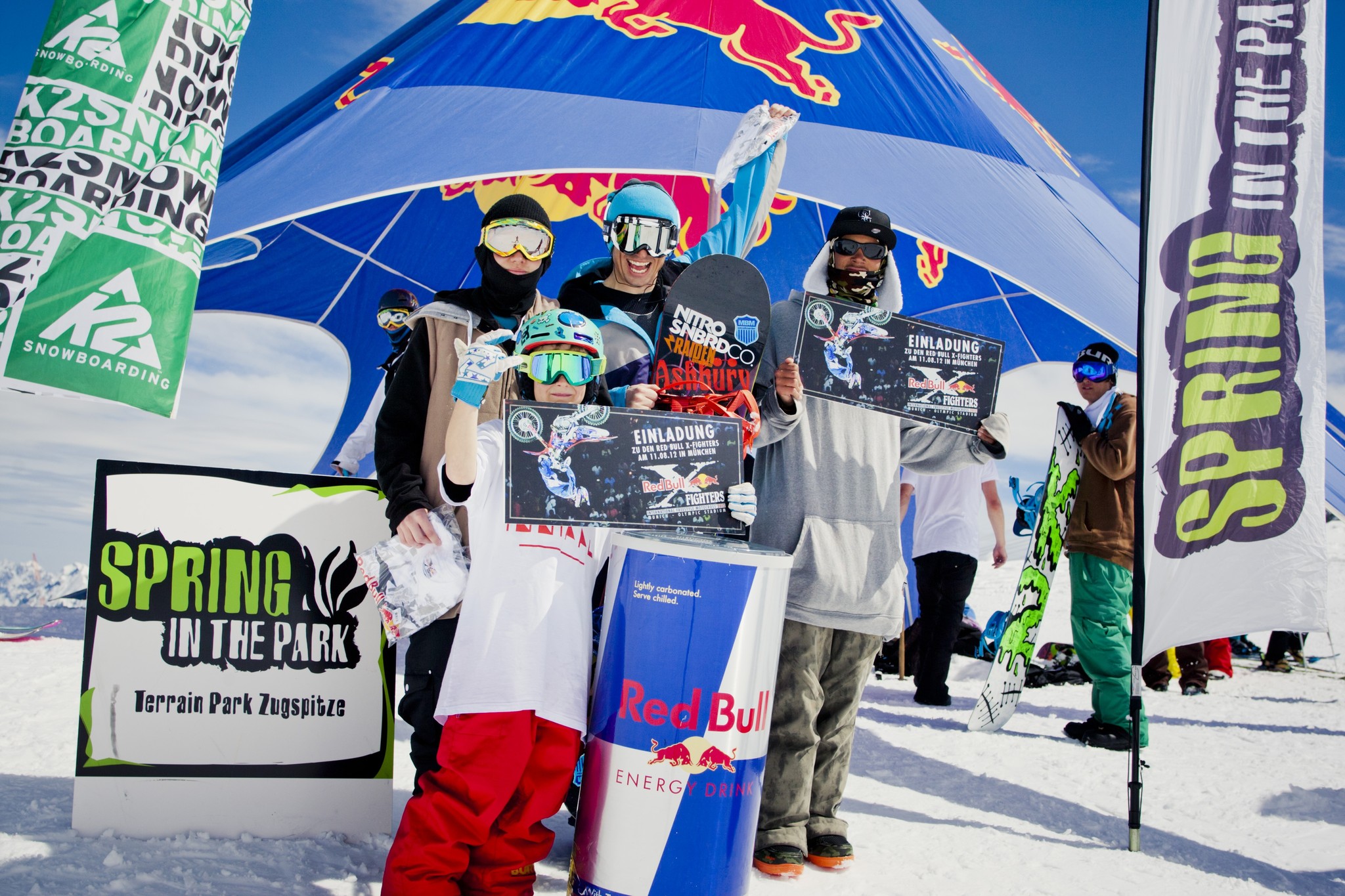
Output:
[804,831,853,868]
[752,846,804,875]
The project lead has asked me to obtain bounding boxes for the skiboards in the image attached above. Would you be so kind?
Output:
[0,619,63,640]
[1231,651,1341,662]
[1233,664,1345,680]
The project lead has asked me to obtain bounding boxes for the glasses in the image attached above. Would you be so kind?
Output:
[832,239,885,259]
[527,349,594,387]
[1072,361,1109,384]
[377,308,410,329]
[482,221,555,261]
[611,216,678,258]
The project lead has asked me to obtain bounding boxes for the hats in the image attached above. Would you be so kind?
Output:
[1078,344,1120,369]
[478,196,553,276]
[606,186,678,232]
[826,206,897,253]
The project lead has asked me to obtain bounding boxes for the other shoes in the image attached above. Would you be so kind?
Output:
[1287,649,1303,663]
[1064,720,1107,740]
[1081,728,1134,748]
[1151,682,1170,692]
[1261,659,1293,673]
[1183,684,1202,696]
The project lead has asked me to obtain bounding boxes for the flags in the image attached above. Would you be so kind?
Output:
[1137,0,1332,668]
[0,0,253,420]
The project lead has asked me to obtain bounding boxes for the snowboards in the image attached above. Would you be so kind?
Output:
[967,406,1086,732]
[564,255,772,819]
[1036,642,1078,660]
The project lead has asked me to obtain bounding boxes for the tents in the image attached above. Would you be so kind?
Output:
[195,0,1345,635]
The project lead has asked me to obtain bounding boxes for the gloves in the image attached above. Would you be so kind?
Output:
[448,327,525,408]
[725,482,758,526]
[1058,400,1104,452]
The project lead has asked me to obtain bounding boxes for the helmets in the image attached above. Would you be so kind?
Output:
[512,306,604,400]
[377,289,420,315]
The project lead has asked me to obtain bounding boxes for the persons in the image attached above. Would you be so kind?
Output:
[746,207,1011,875]
[1257,627,1308,673]
[379,301,757,896]
[375,195,614,795]
[330,289,419,477]
[1140,642,1209,697]
[1057,342,1149,752]
[873,637,904,681]
[899,462,1007,706]
[558,99,800,827]
[1200,635,1261,681]
